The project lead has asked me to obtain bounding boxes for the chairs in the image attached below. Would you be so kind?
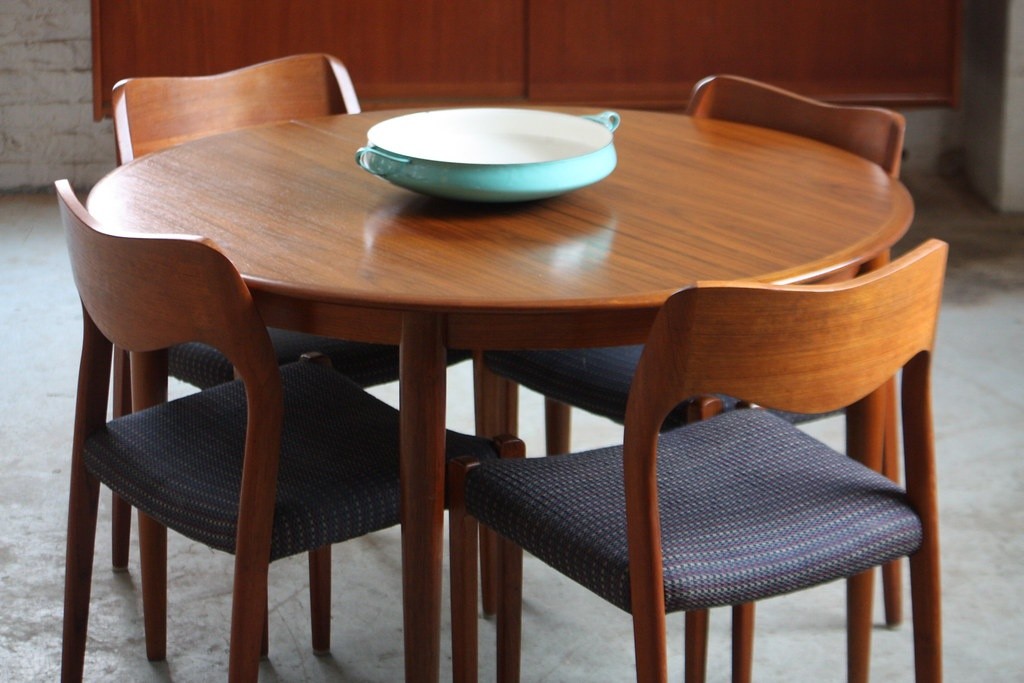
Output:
[56,177,527,683]
[480,74,907,682]
[462,237,951,683]
[112,53,506,656]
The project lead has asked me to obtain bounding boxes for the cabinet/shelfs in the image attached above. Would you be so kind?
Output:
[91,0,966,169]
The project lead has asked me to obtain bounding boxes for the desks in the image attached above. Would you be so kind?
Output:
[85,105,917,682]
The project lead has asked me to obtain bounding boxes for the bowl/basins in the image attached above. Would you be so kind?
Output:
[355,107,620,202]
